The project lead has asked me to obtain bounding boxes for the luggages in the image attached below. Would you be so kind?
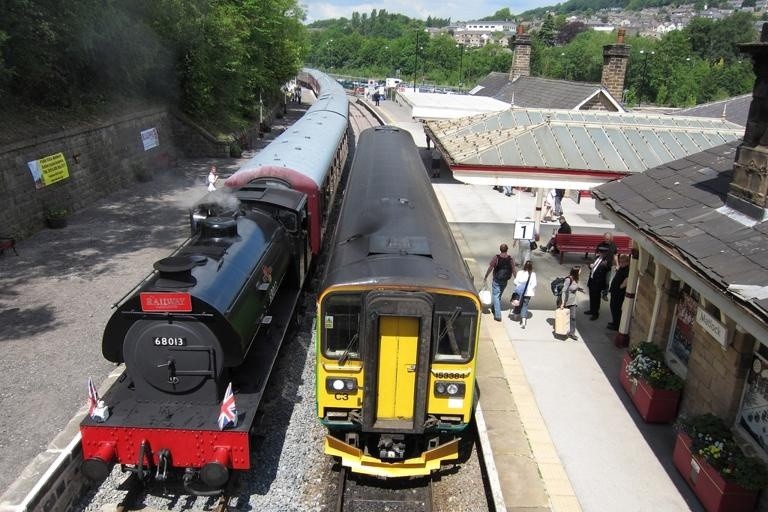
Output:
[554,308,570,335]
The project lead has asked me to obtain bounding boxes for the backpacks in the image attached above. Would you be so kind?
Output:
[493,255,512,281]
[551,276,572,296]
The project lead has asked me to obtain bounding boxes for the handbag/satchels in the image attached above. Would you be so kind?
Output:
[530,241,537,250]
[509,293,520,307]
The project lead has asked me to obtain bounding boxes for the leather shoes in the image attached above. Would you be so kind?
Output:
[606,326,618,330]
[568,335,578,340]
[589,314,599,321]
[602,295,607,301]
[583,310,592,314]
[608,323,613,326]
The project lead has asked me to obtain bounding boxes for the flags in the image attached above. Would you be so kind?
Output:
[87,378,99,420]
[218,382,238,432]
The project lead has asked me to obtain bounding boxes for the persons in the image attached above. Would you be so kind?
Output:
[584,232,630,330]
[514,261,537,329]
[208,166,218,192]
[375,92,380,106]
[483,243,518,321]
[539,217,571,254]
[291,88,301,104]
[432,145,442,178]
[553,189,565,216]
[557,266,585,340]
[513,216,536,268]
[505,186,515,197]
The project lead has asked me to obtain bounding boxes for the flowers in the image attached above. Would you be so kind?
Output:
[675,407,767,494]
[625,340,684,397]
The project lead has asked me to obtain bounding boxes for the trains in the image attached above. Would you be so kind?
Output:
[313,124,484,479]
[78,67,350,497]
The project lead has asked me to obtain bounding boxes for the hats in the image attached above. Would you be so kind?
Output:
[598,247,608,253]
